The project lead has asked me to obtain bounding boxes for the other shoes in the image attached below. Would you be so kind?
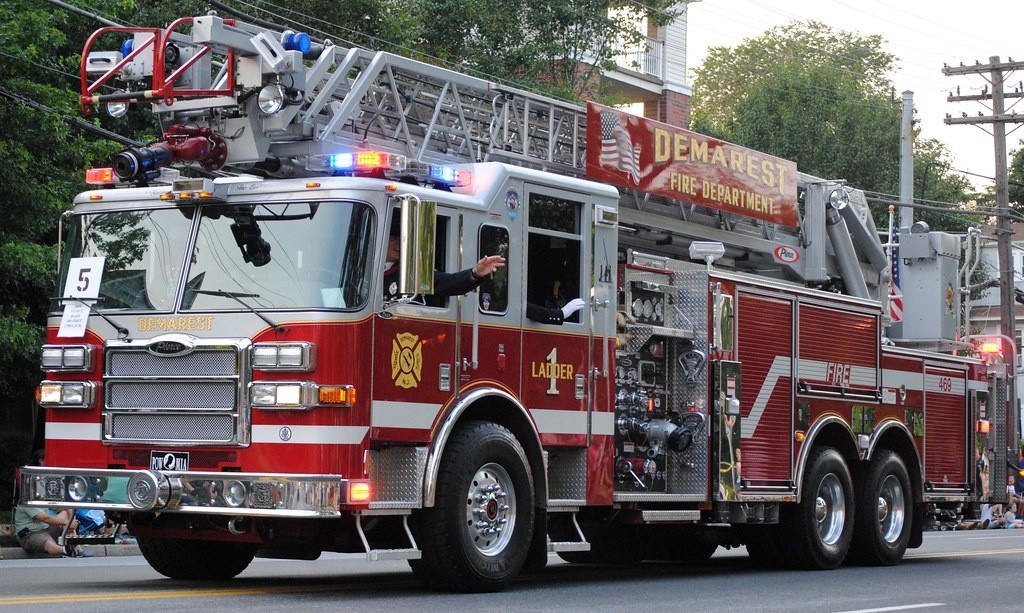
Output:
[966,518,990,530]
[64,546,94,558]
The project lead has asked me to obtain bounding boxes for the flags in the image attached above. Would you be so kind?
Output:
[892,220,902,323]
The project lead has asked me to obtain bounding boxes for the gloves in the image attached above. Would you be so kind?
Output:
[561,298,585,319]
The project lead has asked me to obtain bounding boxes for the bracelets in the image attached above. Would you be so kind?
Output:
[471,268,480,279]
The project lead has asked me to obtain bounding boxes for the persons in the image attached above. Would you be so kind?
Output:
[527,253,585,324]
[14,505,123,557]
[946,447,1024,530]
[383,224,505,300]
[180,480,227,506]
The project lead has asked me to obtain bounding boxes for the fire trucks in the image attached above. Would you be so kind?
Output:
[11,9,1020,592]
